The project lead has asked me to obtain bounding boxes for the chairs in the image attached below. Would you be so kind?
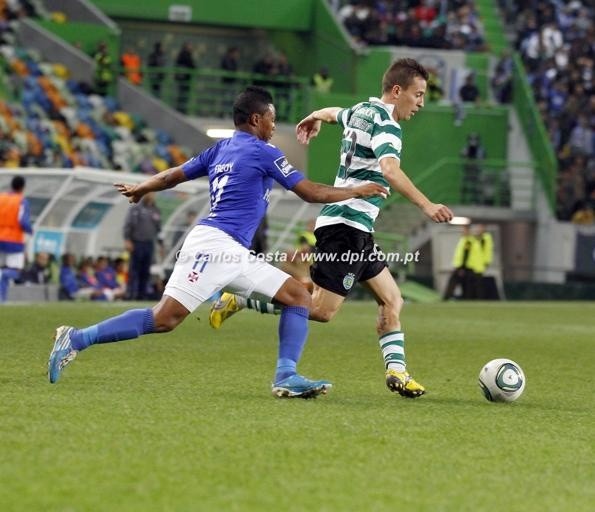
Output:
[1,2,203,185]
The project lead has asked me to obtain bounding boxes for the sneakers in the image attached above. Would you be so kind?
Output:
[271,373,333,400]
[209,291,242,332]
[46,324,79,384]
[384,368,427,398]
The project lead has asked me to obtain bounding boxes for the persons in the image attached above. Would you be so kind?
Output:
[207,57,455,400]
[46,85,391,399]
[0,0,595,305]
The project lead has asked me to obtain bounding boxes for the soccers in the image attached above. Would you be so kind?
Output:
[480,358,525,402]
